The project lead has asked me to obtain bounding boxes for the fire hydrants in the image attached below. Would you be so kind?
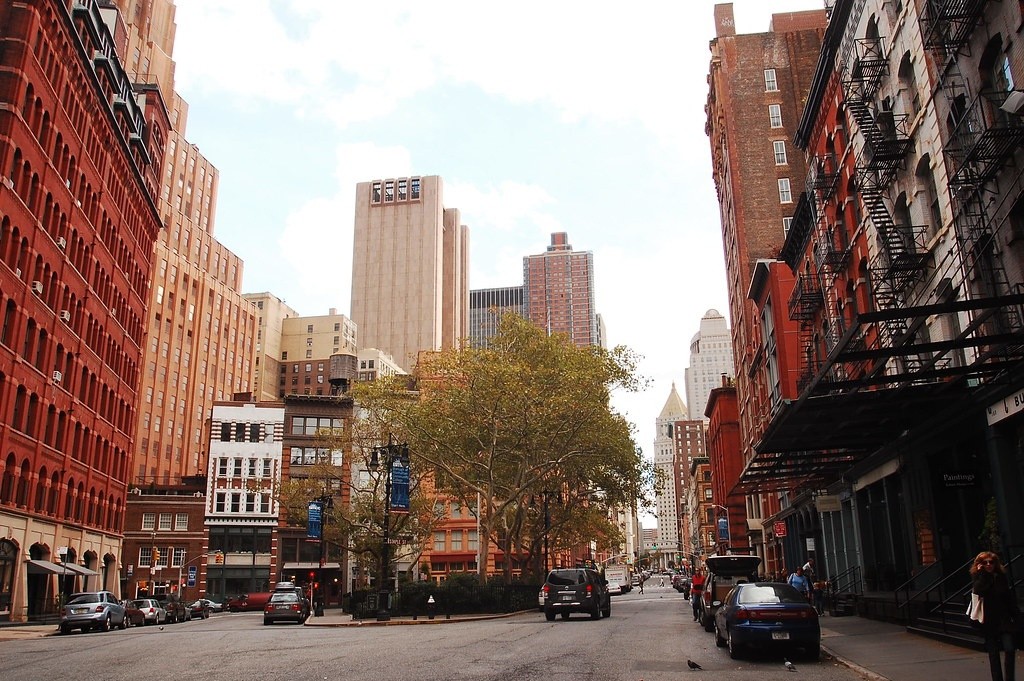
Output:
[427,594,436,619]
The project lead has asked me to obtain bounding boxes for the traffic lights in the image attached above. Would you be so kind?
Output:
[153,550,161,561]
[215,553,223,564]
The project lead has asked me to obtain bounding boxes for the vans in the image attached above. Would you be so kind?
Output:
[538,567,612,622]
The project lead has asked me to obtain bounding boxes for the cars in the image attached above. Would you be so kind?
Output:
[263,581,311,625]
[604,563,652,597]
[663,567,693,600]
[712,581,822,661]
[59,590,128,635]
[118,596,223,629]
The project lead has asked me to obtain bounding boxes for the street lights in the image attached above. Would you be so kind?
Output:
[368,431,412,621]
[711,504,732,552]
[527,487,563,583]
[307,487,334,618]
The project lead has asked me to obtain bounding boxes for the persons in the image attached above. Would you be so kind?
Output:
[812,582,825,617]
[659,577,664,587]
[638,575,645,595]
[689,567,707,623]
[969,552,1017,681]
[759,573,780,583]
[802,558,815,576]
[788,566,810,595]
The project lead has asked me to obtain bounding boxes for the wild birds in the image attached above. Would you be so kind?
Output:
[687,660,702,671]
[784,658,796,671]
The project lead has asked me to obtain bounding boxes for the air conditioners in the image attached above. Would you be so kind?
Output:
[52,370,62,382]
[32,281,43,294]
[112,308,117,316]
[60,309,70,321]
[57,237,66,249]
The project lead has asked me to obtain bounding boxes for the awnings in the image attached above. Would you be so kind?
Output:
[26,559,76,576]
[55,560,101,576]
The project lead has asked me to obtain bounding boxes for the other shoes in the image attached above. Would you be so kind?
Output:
[822,614,825,616]
[818,614,820,617]
[693,616,697,621]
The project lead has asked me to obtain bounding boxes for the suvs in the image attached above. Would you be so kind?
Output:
[693,554,763,633]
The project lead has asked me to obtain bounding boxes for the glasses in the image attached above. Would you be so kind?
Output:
[983,560,995,565]
[694,570,699,572]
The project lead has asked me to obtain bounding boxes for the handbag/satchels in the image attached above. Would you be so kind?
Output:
[966,582,984,624]
[689,597,694,608]
